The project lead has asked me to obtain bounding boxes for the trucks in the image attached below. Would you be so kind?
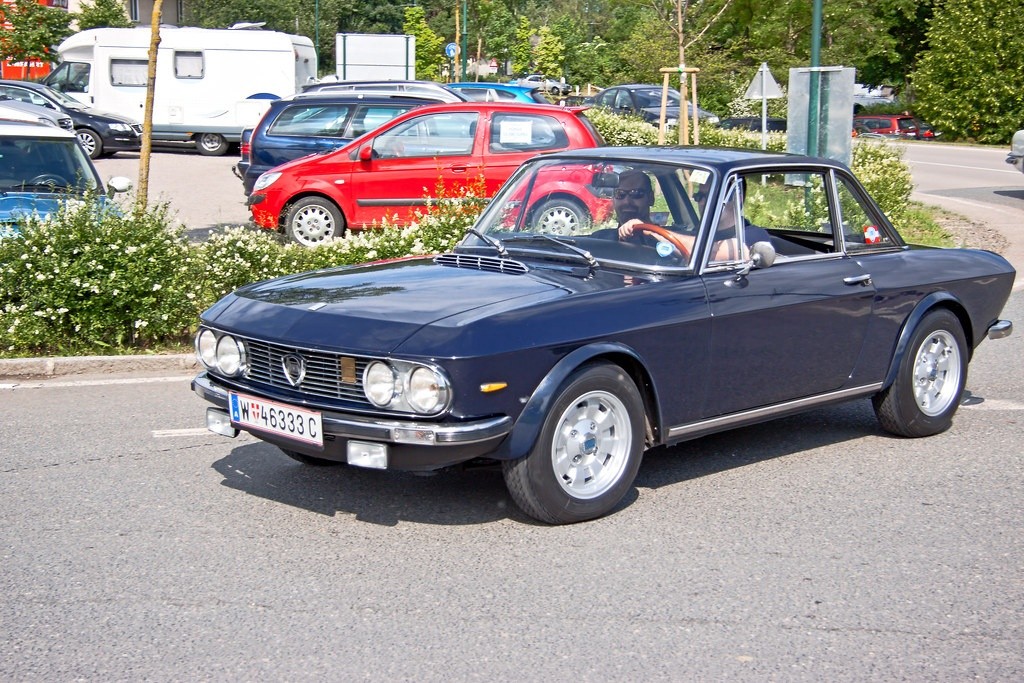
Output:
[44,20,318,157]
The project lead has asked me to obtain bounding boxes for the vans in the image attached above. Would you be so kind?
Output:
[854,114,922,139]
[718,117,787,133]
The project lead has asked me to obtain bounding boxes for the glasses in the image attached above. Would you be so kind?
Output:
[692,193,709,202]
[613,188,651,200]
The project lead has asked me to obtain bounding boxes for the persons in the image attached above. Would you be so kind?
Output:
[619,168,772,264]
[589,171,682,258]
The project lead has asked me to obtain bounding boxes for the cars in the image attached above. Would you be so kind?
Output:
[1006,129,1024,176]
[579,85,720,134]
[519,75,573,97]
[0,79,142,159]
[0,96,77,135]
[246,101,610,252]
[447,79,553,105]
[190,145,1018,527]
[920,121,935,141]
[1,125,134,241]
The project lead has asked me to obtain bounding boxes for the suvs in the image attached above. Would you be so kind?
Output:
[231,78,475,197]
